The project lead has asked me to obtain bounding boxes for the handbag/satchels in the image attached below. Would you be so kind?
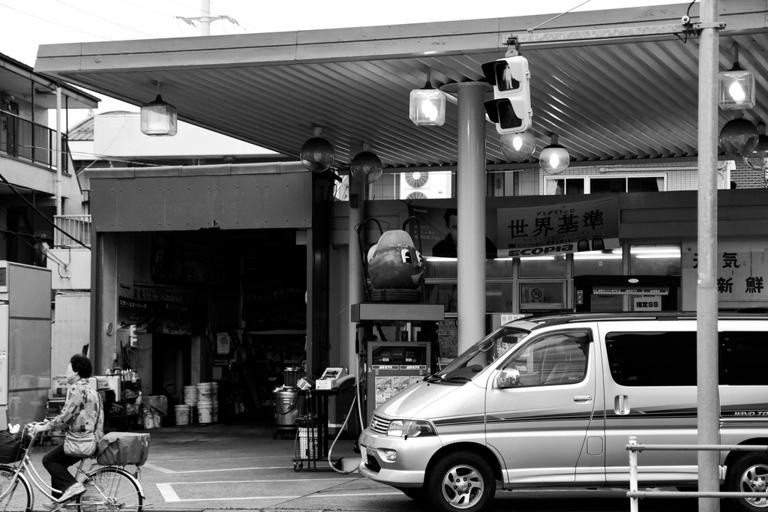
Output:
[63,433,96,456]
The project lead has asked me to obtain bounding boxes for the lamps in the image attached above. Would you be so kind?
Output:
[538,134,571,175]
[349,143,384,185]
[719,118,768,171]
[408,66,446,127]
[718,40,756,111]
[501,131,536,161]
[140,80,177,136]
[299,126,335,173]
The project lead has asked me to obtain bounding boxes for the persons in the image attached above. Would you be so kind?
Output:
[29,231,49,267]
[428,208,498,261]
[30,353,105,511]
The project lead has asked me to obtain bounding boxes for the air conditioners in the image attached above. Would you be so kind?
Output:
[400,172,452,200]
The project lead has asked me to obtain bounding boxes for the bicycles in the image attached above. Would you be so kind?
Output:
[0,420,145,512]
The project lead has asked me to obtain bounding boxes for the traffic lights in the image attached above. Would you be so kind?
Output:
[480,56,533,135]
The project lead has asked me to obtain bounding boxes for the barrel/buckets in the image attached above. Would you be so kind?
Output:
[175,382,219,425]
[275,391,304,425]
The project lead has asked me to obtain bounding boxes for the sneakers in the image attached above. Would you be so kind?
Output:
[43,483,86,509]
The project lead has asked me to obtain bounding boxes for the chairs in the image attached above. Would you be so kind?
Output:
[543,362,585,384]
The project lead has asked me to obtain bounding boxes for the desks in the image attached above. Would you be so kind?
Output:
[292,388,339,472]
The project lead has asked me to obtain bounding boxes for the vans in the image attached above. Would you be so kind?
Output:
[356,311,768,512]
